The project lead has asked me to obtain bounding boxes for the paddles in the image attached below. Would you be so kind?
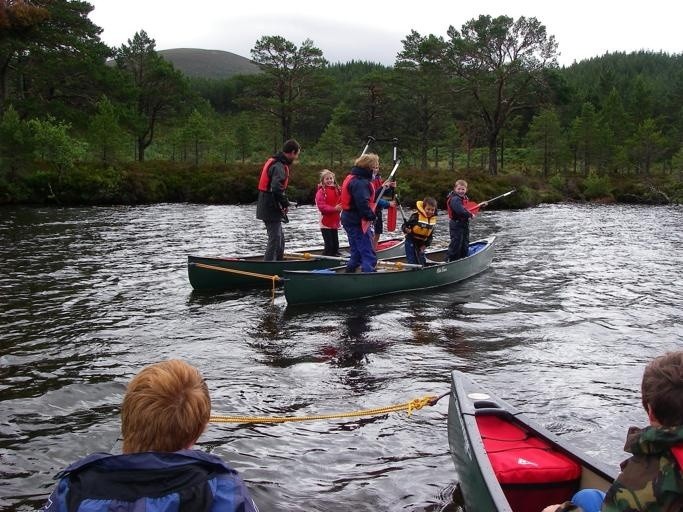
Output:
[465,187,516,213]
[361,159,401,234]
[388,138,399,231]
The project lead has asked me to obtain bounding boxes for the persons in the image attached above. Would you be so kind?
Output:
[252,137,301,264]
[444,179,489,262]
[368,166,396,252]
[313,168,345,257]
[599,346,683,512]
[40,353,257,511]
[399,195,438,267]
[339,152,390,274]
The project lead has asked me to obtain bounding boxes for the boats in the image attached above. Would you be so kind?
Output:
[280,234,497,306]
[447,368,620,512]
[185,233,406,293]
